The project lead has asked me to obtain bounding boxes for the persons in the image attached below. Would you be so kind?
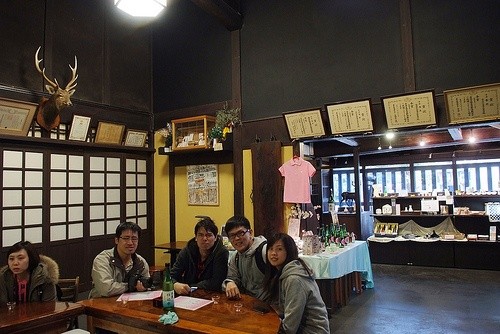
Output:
[222,216,275,302]
[261,233,330,334]
[0,241,59,305]
[88,222,152,299]
[170,219,229,294]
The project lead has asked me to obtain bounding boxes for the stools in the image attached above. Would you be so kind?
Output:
[149,266,164,276]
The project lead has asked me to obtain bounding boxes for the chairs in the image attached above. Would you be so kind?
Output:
[57,276,80,329]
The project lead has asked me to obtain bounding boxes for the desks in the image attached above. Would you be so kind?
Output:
[152,241,189,258]
[296,240,375,315]
[0,288,282,334]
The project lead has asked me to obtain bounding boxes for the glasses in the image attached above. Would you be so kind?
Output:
[228,228,250,239]
[118,236,139,241]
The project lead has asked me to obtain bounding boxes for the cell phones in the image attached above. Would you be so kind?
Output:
[250,305,270,314]
[190,286,198,291]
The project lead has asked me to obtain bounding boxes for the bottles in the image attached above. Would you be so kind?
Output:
[162,263,174,311]
[378,224,397,233]
[303,223,355,255]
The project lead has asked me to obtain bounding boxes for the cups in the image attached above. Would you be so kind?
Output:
[234,303,243,312]
[376,208,382,214]
[212,295,220,304]
[122,296,130,304]
[6,301,16,310]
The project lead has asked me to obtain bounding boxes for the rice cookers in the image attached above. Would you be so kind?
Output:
[381,204,393,215]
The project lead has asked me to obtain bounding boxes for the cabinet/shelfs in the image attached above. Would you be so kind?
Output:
[367,195,500,272]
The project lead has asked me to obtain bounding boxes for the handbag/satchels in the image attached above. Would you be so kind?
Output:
[304,234,319,255]
